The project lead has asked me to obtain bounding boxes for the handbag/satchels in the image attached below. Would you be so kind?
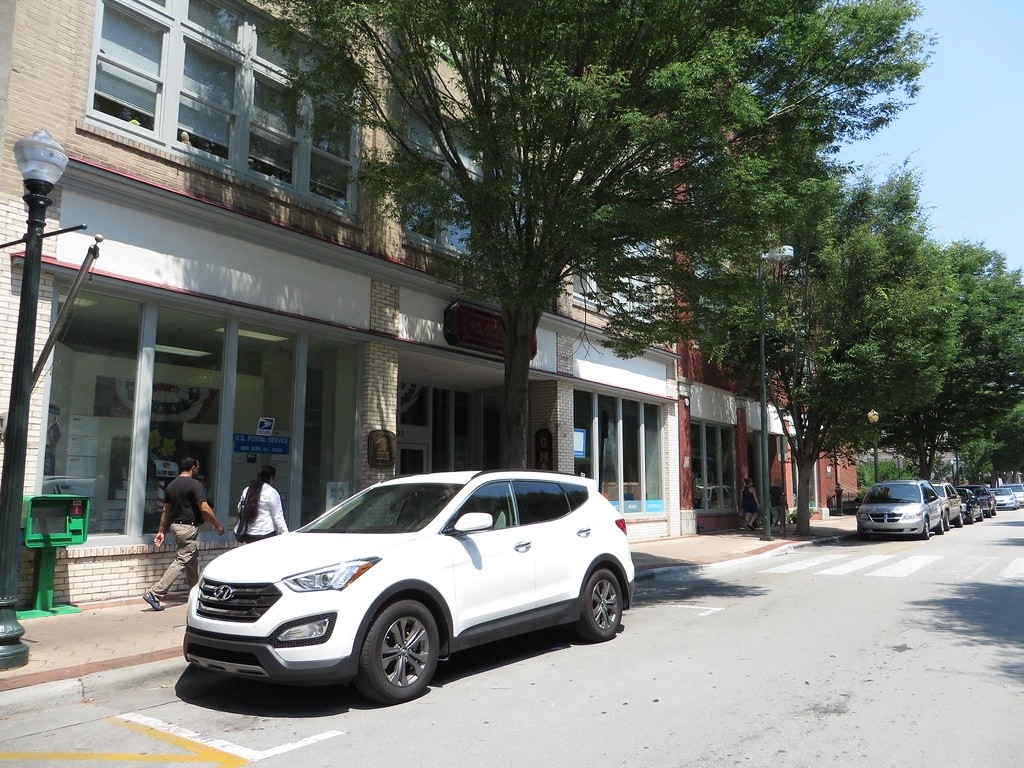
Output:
[234,501,246,543]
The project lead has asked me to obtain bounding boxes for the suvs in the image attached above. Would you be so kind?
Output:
[1000,484,1024,507]
[181,466,636,704]
[42,473,97,521]
[958,485,997,518]
[856,478,944,540]
[929,480,963,530]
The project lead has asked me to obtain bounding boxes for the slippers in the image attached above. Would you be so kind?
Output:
[747,525,755,531]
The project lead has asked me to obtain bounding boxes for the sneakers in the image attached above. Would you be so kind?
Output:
[143,592,165,610]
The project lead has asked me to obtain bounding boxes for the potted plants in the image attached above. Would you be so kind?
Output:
[789,508,814,525]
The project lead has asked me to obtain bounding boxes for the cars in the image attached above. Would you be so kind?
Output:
[988,488,1019,509]
[954,488,984,524]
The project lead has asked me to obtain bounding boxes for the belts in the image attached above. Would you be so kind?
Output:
[175,521,196,526]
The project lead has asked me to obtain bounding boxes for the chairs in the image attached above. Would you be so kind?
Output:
[394,487,452,528]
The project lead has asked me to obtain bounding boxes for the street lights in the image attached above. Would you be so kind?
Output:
[758,245,795,538]
[867,408,881,482]
[0,126,72,669]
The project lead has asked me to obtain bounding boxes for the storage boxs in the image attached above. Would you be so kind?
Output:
[257,430,289,521]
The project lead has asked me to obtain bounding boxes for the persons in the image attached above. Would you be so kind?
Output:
[143,456,225,611]
[232,465,289,544]
[769,478,788,535]
[740,477,760,531]
